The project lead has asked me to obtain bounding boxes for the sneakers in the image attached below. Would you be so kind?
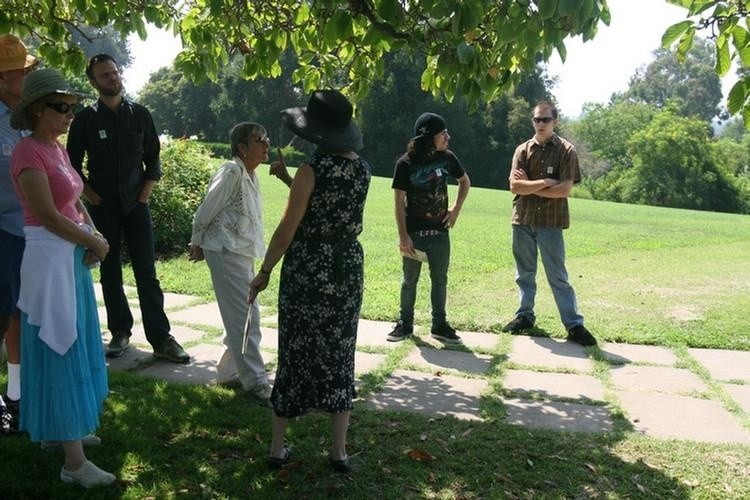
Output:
[502,313,534,334]
[60,460,117,488]
[153,333,191,364]
[38,432,103,447]
[567,324,597,348]
[104,330,132,359]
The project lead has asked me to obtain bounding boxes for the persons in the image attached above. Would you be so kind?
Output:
[502,101,598,347]
[10,67,117,489]
[0,33,40,436]
[189,121,273,401]
[66,53,192,363]
[246,90,370,473]
[386,112,471,344]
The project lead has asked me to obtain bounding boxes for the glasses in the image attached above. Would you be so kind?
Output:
[533,116,555,123]
[5,65,34,75]
[40,101,77,114]
[247,136,271,145]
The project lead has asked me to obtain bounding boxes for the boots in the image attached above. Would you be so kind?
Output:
[429,316,461,344]
[385,318,414,343]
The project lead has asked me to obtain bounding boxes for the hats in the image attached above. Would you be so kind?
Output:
[9,68,96,131]
[412,112,447,140]
[279,89,364,153]
[0,33,40,72]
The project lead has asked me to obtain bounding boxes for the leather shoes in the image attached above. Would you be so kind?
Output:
[330,453,352,475]
[267,446,297,468]
[251,382,275,401]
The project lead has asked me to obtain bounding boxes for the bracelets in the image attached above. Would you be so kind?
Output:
[259,264,271,277]
